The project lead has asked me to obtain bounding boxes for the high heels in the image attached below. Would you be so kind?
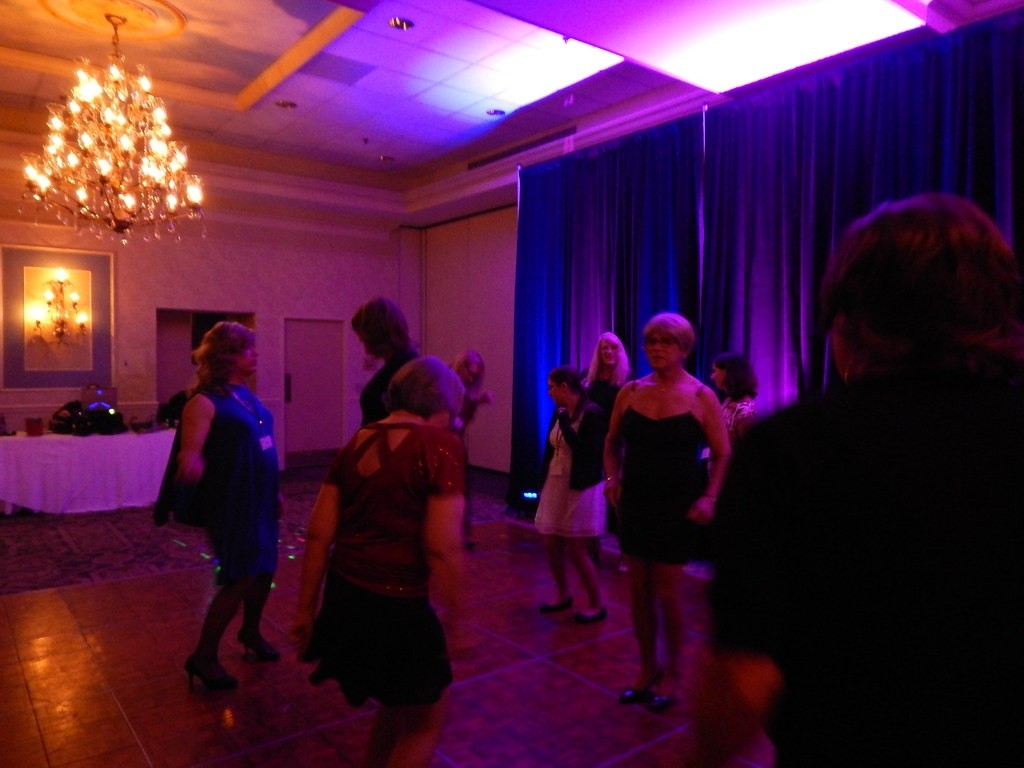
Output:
[238,632,280,660]
[185,656,238,691]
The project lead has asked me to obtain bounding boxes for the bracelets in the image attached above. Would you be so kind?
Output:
[608,477,610,480]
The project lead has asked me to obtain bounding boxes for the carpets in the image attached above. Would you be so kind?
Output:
[0,461,508,595]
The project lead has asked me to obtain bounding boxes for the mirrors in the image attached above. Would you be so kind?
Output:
[0,250,117,393]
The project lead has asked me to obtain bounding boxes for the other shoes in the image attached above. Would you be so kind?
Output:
[617,668,663,702]
[538,596,572,612]
[650,670,681,710]
[572,605,608,623]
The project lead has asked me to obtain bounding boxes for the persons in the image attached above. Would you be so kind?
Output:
[351,297,415,427]
[174,321,281,688]
[659,196,1023,767]
[708,354,757,479]
[534,366,611,622]
[581,333,630,413]
[602,313,730,709]
[445,350,489,547]
[289,356,473,768]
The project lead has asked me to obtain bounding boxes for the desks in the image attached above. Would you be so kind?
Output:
[0,423,176,519]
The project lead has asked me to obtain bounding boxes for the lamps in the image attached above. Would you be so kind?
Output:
[26,261,91,348]
[22,11,208,247]
